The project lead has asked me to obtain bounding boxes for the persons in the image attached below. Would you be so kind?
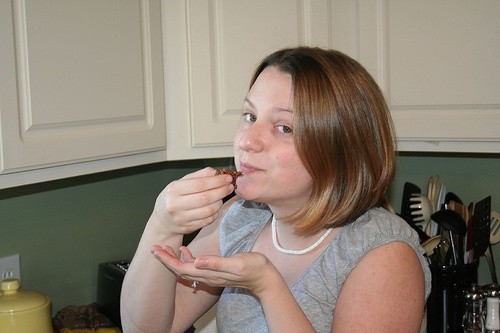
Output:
[120,47,432,333]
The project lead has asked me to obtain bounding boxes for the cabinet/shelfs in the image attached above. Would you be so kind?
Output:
[0,0,500,189]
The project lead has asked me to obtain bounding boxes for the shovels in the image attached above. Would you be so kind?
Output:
[471,194,492,261]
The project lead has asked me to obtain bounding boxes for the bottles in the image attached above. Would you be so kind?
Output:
[461,283,488,333]
[485,284,500,331]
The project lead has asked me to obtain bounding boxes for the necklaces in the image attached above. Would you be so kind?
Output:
[271,214,333,255]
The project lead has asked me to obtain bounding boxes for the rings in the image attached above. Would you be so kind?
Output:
[192,280,198,288]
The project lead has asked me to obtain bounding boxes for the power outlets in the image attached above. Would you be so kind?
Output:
[0,253,21,288]
